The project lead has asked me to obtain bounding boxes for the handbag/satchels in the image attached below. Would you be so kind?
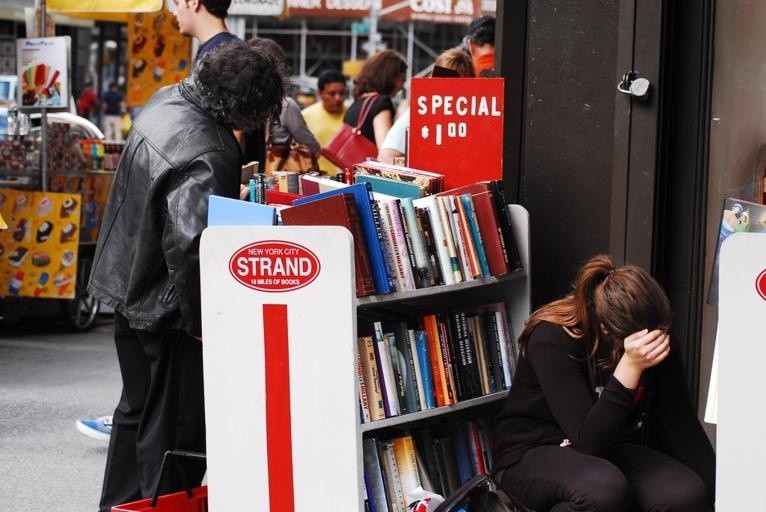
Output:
[321,123,378,172]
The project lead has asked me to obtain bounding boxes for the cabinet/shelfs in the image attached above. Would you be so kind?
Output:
[198,201,531,512]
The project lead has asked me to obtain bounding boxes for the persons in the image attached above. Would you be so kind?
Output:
[77,78,99,126]
[76,0,265,441]
[86,38,285,512]
[102,81,127,141]
[490,255,711,512]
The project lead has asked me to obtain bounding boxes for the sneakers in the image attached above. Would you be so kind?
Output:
[76,416,113,441]
